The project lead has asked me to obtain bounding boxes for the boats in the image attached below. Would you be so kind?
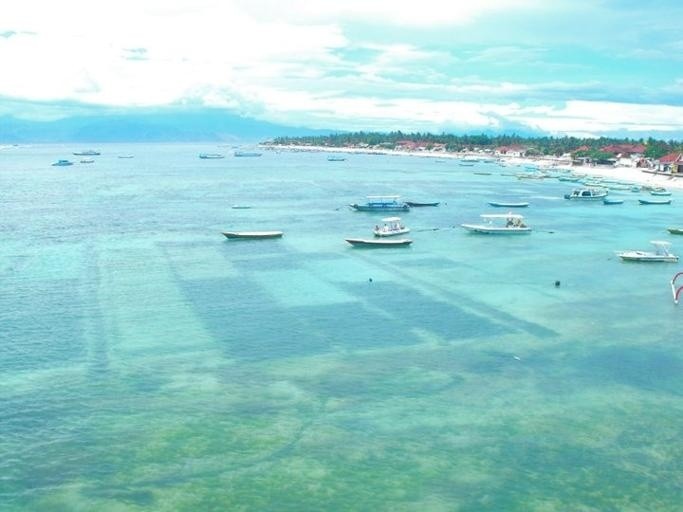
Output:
[371,215,412,238]
[341,236,414,249]
[326,156,345,161]
[220,230,284,240]
[230,204,252,210]
[432,154,683,266]
[46,149,135,168]
[406,201,441,208]
[350,194,409,213]
[198,142,281,159]
